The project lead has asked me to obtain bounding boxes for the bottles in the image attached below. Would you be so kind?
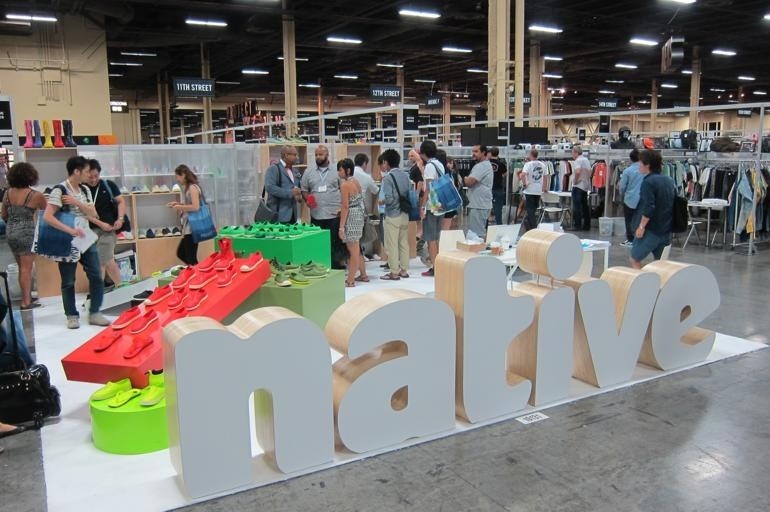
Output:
[306,192,318,208]
[499,246,505,255]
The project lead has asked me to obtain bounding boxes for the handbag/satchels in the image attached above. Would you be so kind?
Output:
[184,203,217,245]
[1,351,61,425]
[30,208,82,265]
[361,219,378,245]
[427,173,470,216]
[397,196,412,212]
[670,188,688,233]
[252,198,279,227]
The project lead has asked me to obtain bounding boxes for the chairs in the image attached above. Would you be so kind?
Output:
[486,223,523,290]
[532,222,562,280]
[673,205,703,249]
[438,229,466,254]
[539,192,571,228]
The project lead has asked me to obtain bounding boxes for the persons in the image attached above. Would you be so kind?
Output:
[570,146,592,232]
[263,139,507,289]
[0,154,10,202]
[80,158,126,309]
[610,125,637,150]
[2,162,48,309]
[618,148,646,248]
[628,149,677,270]
[0,417,30,456]
[42,156,111,330]
[519,148,550,230]
[165,164,210,268]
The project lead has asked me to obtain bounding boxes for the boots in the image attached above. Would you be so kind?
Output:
[22,119,78,148]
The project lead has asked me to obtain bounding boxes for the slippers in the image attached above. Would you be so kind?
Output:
[379,273,400,281]
[397,271,409,278]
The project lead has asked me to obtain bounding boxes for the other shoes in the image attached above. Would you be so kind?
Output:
[269,256,326,287]
[223,222,322,239]
[91,377,164,408]
[92,249,264,359]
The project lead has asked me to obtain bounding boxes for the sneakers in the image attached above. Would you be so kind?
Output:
[19,302,43,312]
[118,183,181,196]
[568,224,591,232]
[66,313,81,330]
[420,268,435,277]
[618,239,636,249]
[30,296,40,304]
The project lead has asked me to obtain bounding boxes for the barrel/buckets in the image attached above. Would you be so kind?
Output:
[542,206,563,223]
[612,216,626,236]
[599,217,614,236]
[6,262,35,301]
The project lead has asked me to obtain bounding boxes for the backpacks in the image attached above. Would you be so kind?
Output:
[711,136,741,152]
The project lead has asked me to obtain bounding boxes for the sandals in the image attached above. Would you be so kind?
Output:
[354,275,369,282]
[344,280,356,288]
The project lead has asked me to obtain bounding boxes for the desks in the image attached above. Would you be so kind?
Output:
[686,201,727,246]
[480,238,608,279]
[546,190,591,231]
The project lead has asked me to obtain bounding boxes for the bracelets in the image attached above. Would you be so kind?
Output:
[638,222,647,229]
[522,184,526,187]
[117,215,124,221]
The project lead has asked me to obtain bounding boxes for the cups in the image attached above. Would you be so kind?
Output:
[501,239,511,249]
[490,241,500,256]
